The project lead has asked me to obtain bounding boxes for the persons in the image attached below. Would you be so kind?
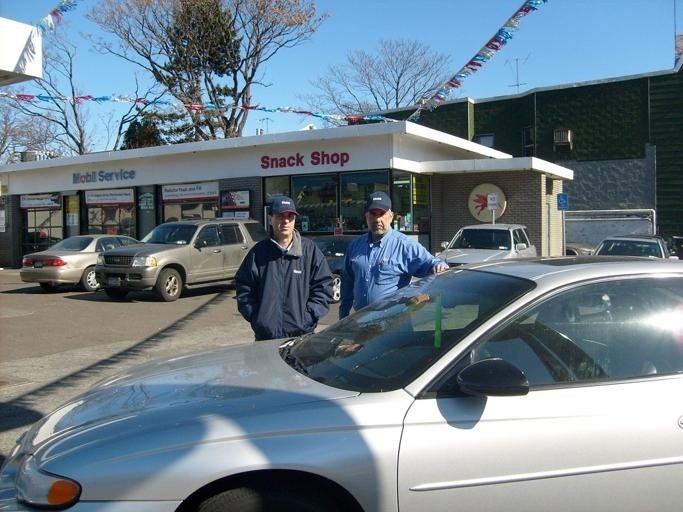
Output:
[234,194,334,341]
[338,191,450,320]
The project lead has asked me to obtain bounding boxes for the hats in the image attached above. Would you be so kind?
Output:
[268,196,302,216]
[364,191,392,213]
